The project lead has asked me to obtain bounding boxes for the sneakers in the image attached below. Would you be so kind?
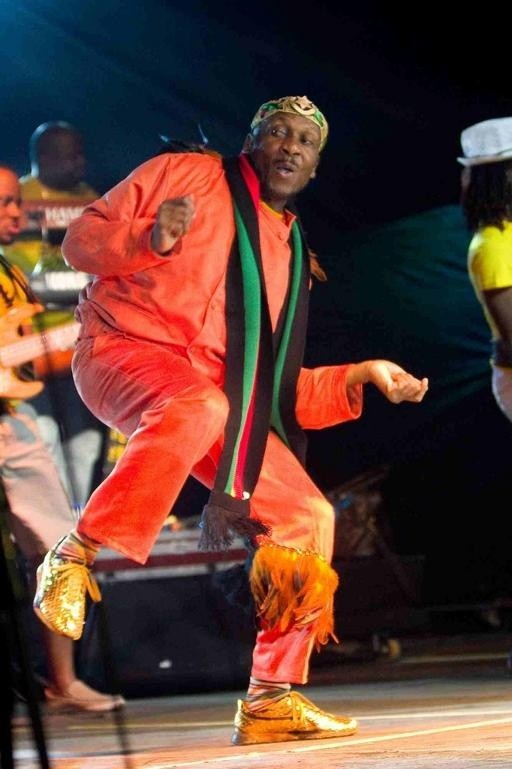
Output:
[32,535,103,640]
[231,690,357,745]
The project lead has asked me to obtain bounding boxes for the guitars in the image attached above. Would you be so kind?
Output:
[0,303,82,399]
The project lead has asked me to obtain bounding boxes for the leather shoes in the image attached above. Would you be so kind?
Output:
[45,679,125,711]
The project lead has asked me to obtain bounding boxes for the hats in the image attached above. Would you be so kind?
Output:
[456,116,512,166]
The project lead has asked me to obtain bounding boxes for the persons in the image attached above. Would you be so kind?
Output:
[1,165,126,727]
[457,116,511,423]
[2,117,109,503]
[32,94,431,747]
